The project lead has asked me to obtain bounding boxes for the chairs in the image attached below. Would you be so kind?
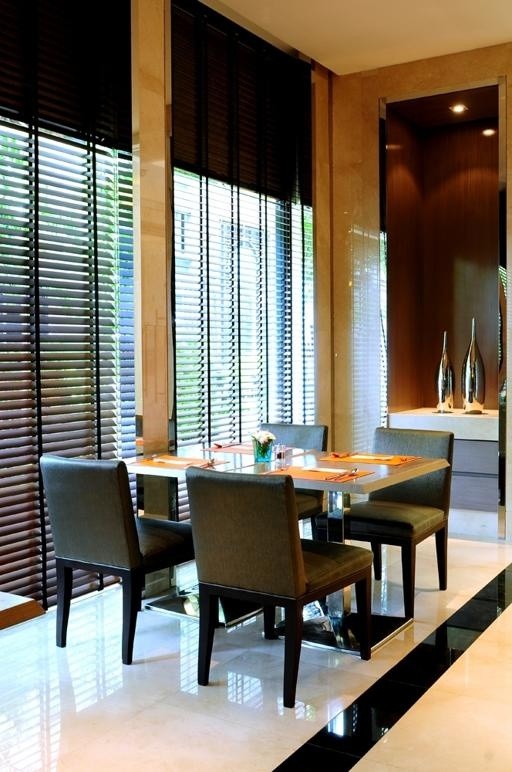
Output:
[38,449,196,666]
[183,464,375,709]
[339,426,457,622]
[254,421,327,543]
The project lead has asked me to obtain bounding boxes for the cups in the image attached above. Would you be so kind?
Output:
[274,445,285,470]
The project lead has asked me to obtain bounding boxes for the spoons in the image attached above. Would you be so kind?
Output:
[325,468,358,481]
[214,443,243,449]
[200,458,216,469]
